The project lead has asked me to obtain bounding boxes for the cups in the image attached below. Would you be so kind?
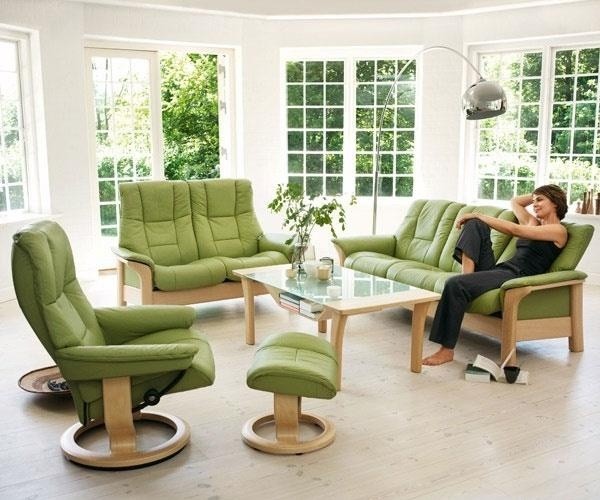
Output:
[327,286,342,299]
[285,270,298,280]
[316,266,329,280]
[504,366,520,384]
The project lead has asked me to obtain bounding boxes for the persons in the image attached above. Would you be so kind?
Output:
[420,185,568,365]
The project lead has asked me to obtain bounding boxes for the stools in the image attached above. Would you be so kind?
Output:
[240,331,340,457]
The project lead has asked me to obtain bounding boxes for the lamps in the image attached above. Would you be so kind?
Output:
[370,45,506,240]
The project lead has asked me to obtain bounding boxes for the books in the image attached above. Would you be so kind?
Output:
[278,291,324,318]
[464,348,528,384]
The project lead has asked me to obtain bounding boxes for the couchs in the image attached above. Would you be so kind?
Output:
[109,177,293,305]
[10,219,216,471]
[331,199,594,367]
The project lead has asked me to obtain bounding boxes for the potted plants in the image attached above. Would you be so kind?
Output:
[266,180,358,275]
